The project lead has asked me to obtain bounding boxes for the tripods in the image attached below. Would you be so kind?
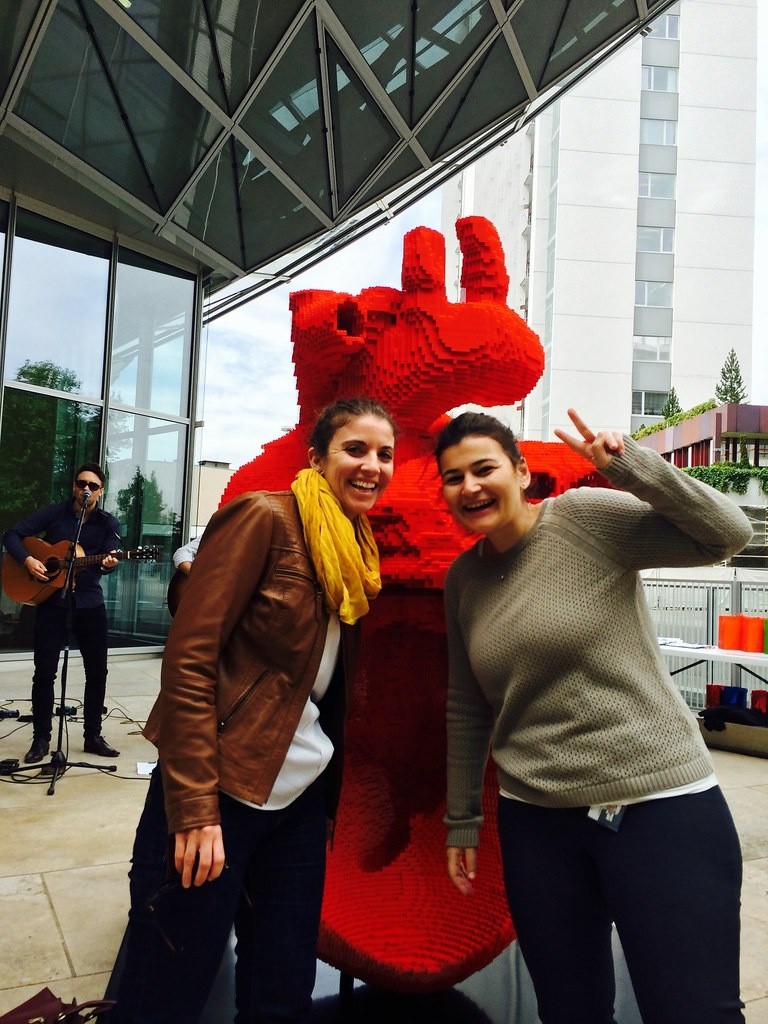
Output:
[0,499,117,796]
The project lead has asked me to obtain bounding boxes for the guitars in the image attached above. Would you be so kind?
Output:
[166,570,187,621]
[1,536,160,607]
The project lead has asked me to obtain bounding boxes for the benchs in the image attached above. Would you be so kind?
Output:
[696,716,768,759]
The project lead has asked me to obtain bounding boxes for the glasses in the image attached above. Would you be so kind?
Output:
[74,479,103,491]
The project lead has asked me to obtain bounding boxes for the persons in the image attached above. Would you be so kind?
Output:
[171,532,202,573]
[598,806,618,827]
[2,462,122,763]
[94,395,397,1023]
[434,411,755,1024]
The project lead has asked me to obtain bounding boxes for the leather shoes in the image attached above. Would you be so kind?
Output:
[82,735,121,758]
[24,735,51,764]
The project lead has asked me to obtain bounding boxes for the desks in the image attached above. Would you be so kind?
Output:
[659,645,768,687]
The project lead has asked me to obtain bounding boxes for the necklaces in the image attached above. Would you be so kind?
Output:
[500,501,531,581]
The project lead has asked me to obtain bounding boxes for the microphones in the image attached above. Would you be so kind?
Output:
[83,490,92,504]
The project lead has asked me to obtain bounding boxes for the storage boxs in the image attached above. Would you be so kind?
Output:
[720,686,748,709]
[751,689,768,713]
[706,684,727,709]
[741,616,764,653]
[718,615,741,650]
[764,618,768,655]
